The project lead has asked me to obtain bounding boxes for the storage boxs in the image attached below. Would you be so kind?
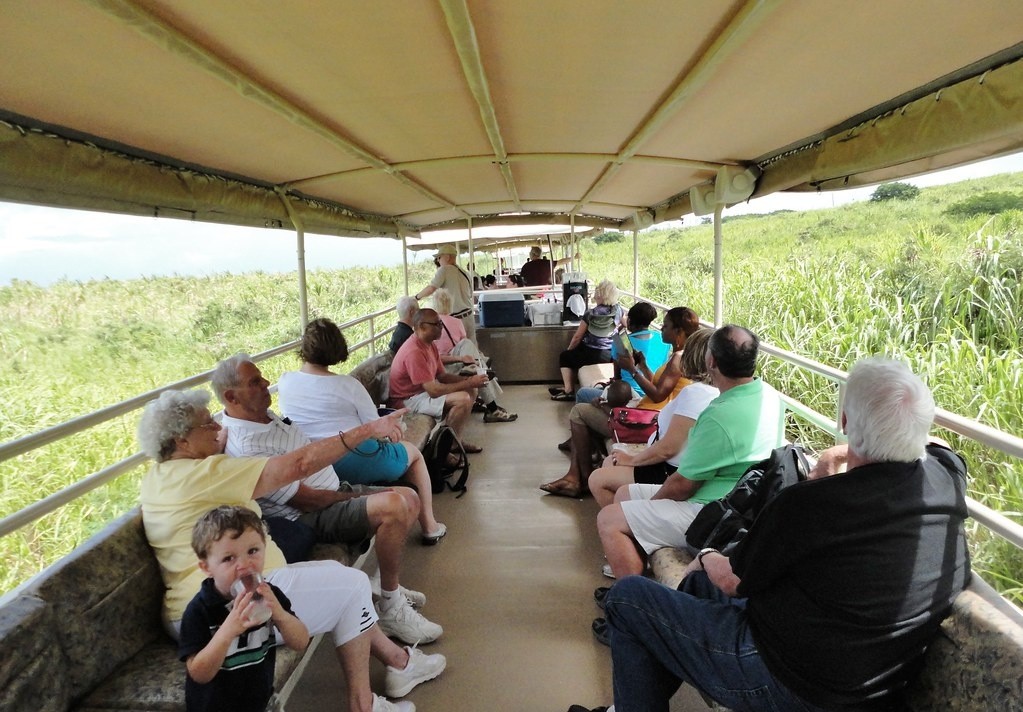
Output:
[527,303,562,325]
[478,291,525,328]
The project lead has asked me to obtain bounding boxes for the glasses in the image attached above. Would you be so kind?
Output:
[438,254,448,259]
[185,414,216,433]
[419,320,444,330]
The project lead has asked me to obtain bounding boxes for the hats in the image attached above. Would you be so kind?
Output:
[432,244,457,257]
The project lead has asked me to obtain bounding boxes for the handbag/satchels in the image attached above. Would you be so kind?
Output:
[375,406,405,441]
[607,406,660,444]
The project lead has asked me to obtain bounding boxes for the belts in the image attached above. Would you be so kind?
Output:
[455,309,473,319]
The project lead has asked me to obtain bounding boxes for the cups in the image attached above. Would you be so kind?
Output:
[612,443,628,455]
[475,368,487,377]
[230,568,272,624]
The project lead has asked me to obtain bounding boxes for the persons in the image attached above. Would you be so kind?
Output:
[591,324,786,647]
[591,380,643,408]
[568,355,973,712]
[210,353,443,644]
[558,303,672,453]
[178,505,309,712]
[137,388,447,712]
[467,262,498,290]
[389,296,518,423]
[506,274,531,300]
[432,288,487,413]
[520,246,581,286]
[414,243,478,349]
[548,280,624,402]
[278,319,446,546]
[539,307,699,498]
[389,309,489,470]
[588,328,720,580]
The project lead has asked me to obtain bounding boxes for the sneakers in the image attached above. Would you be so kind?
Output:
[375,593,444,644]
[593,587,611,609]
[458,364,496,382]
[483,405,519,423]
[591,617,612,646]
[370,571,426,608]
[384,638,447,697]
[371,693,416,712]
[548,385,576,399]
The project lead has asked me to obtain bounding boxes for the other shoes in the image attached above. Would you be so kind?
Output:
[559,436,572,452]
[592,450,603,464]
[567,703,609,712]
[419,522,447,545]
[449,441,483,454]
[473,398,488,413]
[601,563,616,578]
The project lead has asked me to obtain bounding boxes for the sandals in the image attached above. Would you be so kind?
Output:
[540,477,581,496]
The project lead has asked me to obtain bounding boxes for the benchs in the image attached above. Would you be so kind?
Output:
[578,363,1023,712]
[0,352,436,712]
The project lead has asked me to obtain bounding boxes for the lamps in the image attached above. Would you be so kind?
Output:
[634,210,655,229]
[690,185,721,216]
[714,165,761,204]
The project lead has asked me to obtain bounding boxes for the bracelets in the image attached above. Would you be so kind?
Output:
[415,294,420,300]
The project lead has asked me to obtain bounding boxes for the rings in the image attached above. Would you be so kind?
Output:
[484,382,485,384]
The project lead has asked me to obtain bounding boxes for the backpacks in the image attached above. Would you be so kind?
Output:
[686,443,815,557]
[422,424,469,498]
